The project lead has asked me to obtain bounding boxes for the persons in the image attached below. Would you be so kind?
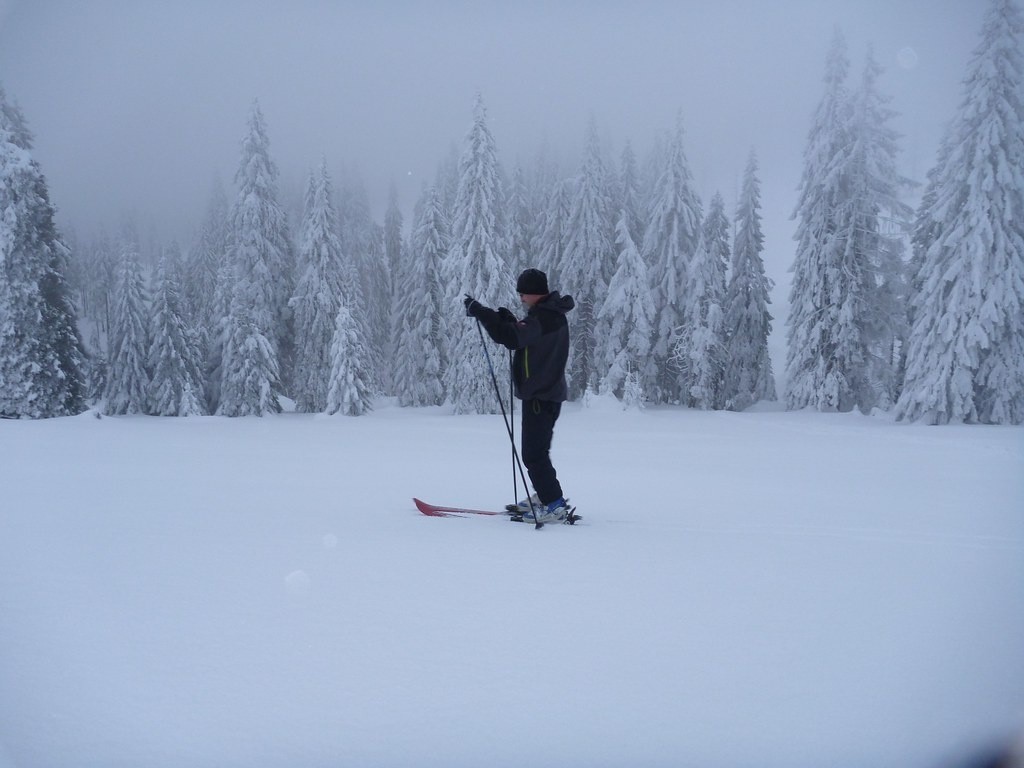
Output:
[463,268,575,525]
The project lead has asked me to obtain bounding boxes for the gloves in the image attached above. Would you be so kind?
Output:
[463,293,488,317]
[496,307,517,323]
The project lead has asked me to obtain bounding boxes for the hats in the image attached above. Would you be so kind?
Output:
[516,269,548,295]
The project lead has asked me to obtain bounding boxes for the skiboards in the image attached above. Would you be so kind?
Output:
[414,498,583,525]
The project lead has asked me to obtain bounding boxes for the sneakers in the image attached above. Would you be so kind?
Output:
[517,493,543,508]
[522,496,567,523]
